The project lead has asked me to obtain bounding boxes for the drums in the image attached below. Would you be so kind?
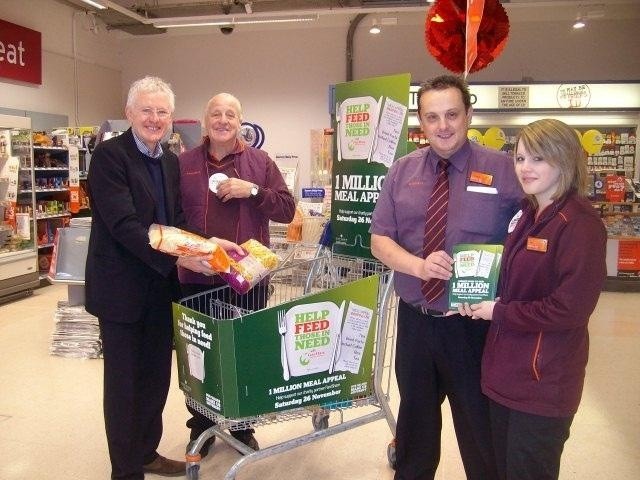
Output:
[329,299,347,375]
[474,249,482,278]
[367,95,384,165]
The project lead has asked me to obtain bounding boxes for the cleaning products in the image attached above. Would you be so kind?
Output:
[172,220,406,479]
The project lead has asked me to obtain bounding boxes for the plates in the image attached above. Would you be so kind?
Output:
[16,144,69,253]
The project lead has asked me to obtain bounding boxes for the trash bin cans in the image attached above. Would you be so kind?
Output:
[249,183,258,200]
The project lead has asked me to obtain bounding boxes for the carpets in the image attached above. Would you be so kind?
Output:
[421,159,452,304]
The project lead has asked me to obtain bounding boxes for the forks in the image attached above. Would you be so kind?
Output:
[453,253,459,279]
[334,102,344,163]
[276,309,291,384]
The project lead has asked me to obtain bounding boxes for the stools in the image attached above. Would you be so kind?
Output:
[406,303,459,317]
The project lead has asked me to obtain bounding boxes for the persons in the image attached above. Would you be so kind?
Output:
[81,73,246,480]
[366,74,525,479]
[176,91,297,460]
[457,117,610,479]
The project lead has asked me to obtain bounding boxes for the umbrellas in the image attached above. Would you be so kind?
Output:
[186,436,215,459]
[232,434,259,456]
[143,455,186,477]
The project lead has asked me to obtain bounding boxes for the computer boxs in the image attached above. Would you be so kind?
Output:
[218,24,235,35]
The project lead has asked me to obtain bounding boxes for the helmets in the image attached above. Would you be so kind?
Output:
[284,301,342,377]
[457,250,481,279]
[338,96,380,160]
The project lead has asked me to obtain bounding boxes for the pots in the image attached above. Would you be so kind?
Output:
[186,342,206,384]
